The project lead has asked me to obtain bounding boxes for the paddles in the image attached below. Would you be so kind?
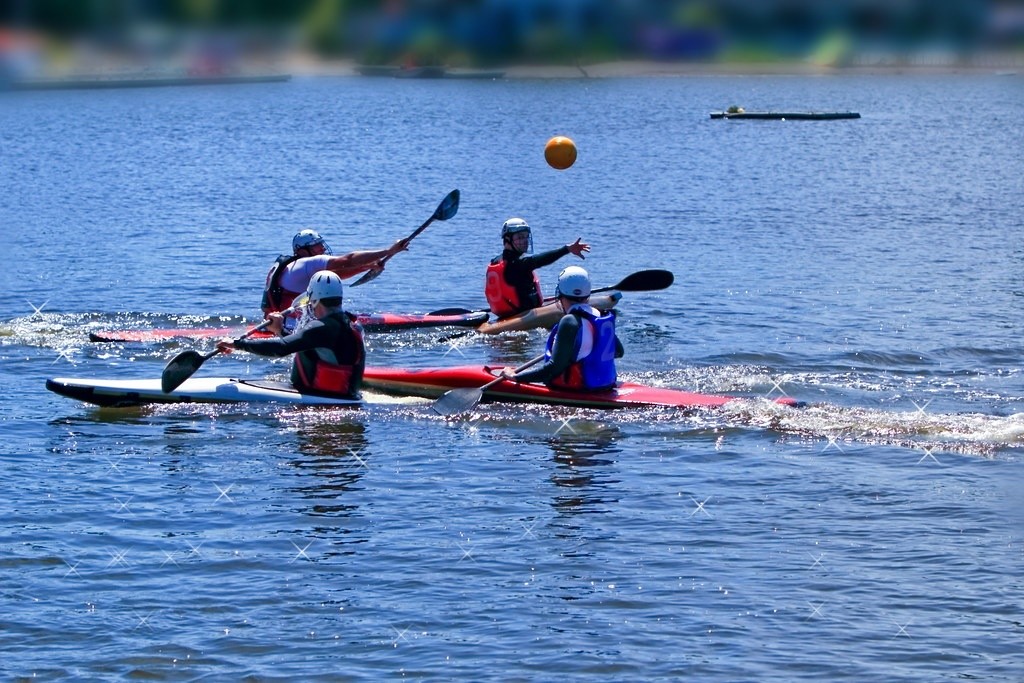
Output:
[428,269,675,317]
[348,189,464,298]
[161,302,308,395]
[431,352,548,416]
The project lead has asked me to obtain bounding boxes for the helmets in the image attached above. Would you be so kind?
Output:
[557,266,591,302]
[500,218,533,254]
[291,229,333,256]
[307,270,344,301]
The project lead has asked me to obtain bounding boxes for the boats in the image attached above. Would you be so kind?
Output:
[45,377,432,406]
[88,312,490,342]
[362,365,807,411]
[475,291,621,335]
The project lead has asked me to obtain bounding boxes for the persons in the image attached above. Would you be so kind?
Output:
[261,229,410,335]
[215,270,367,397]
[499,266,624,392]
[485,218,591,318]
